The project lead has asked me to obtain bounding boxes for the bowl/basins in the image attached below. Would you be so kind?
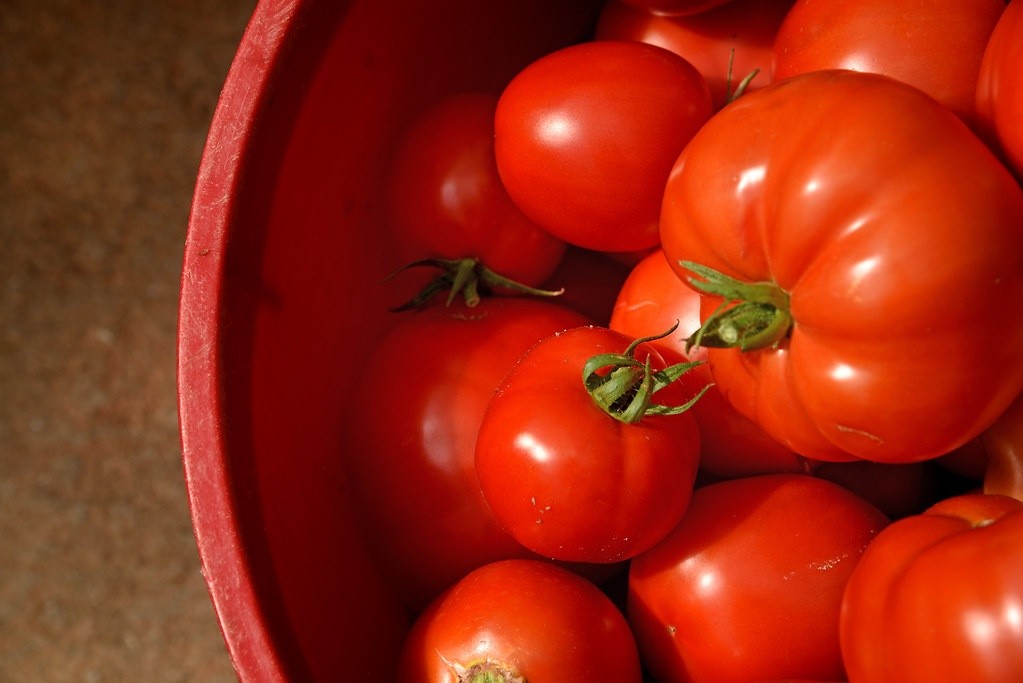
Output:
[179,0,1023,683]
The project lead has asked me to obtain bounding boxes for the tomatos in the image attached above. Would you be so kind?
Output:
[346,0,1023,683]
